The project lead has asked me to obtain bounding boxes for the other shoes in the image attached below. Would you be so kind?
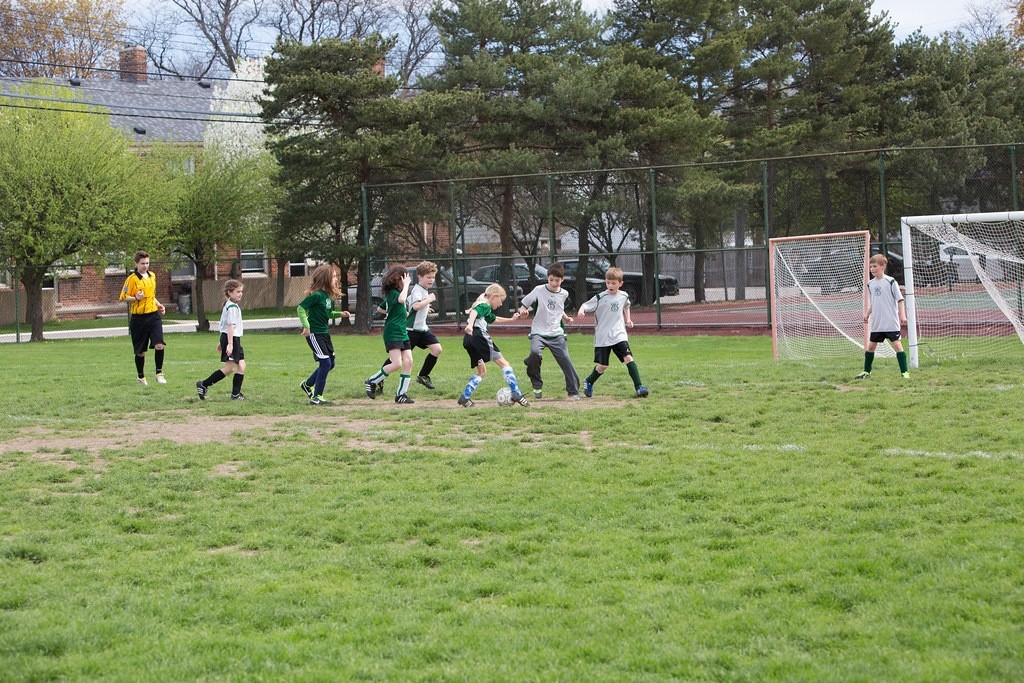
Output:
[156,375,166,383]
[137,376,148,385]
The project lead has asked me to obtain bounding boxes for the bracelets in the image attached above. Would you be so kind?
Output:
[134,296,140,301]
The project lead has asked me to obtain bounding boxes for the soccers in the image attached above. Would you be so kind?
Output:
[496,387,515,406]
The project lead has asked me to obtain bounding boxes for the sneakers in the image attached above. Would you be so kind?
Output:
[901,372,910,379]
[533,389,542,399]
[854,371,871,380]
[458,394,476,408]
[300,380,313,399]
[636,386,648,397]
[416,375,435,389]
[376,379,385,396]
[310,395,329,405]
[365,377,376,399]
[573,394,581,401]
[231,393,245,401]
[196,381,207,399]
[395,394,414,403]
[511,392,531,408]
[583,376,593,397]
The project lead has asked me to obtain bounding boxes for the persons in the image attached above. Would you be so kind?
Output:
[364,265,414,403]
[119,251,167,384]
[297,264,351,405]
[578,267,649,397]
[518,263,580,398]
[458,283,531,408]
[854,254,910,379]
[408,261,442,389]
[195,279,247,401]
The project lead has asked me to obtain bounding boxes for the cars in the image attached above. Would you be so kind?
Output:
[334,267,522,319]
[929,243,1022,282]
[801,248,935,292]
[545,258,679,306]
[459,264,606,313]
